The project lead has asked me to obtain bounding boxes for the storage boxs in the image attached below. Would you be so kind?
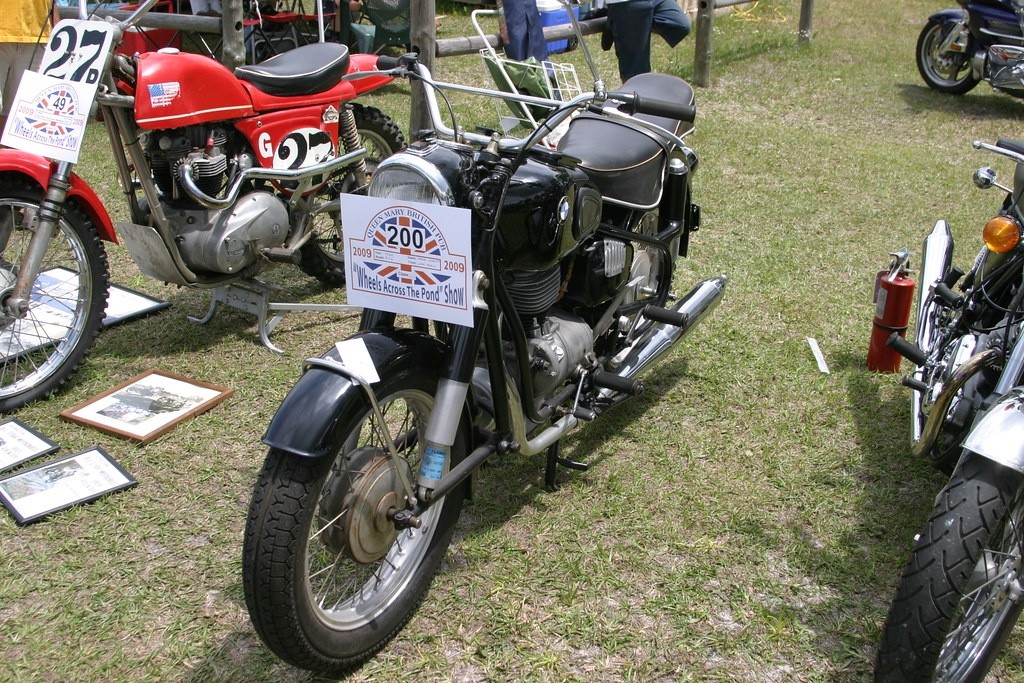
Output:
[539,7,581,53]
[115,25,180,57]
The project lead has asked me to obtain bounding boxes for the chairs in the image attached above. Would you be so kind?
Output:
[244,0,411,64]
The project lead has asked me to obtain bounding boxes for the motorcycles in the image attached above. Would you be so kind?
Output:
[874,138,1023,683]
[915,0,1024,99]
[241,52,731,674]
[0,0,413,417]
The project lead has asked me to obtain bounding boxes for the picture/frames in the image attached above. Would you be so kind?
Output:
[0,417,61,474]
[0,265,174,368]
[0,259,21,290]
[0,446,139,526]
[60,367,234,445]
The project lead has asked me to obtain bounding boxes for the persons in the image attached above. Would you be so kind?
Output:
[0,0,61,148]
[328,0,446,41]
[601,0,691,84]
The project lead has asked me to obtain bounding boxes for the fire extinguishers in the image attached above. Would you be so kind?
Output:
[867,248,917,374]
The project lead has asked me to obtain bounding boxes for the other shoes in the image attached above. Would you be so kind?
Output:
[601,17,613,51]
